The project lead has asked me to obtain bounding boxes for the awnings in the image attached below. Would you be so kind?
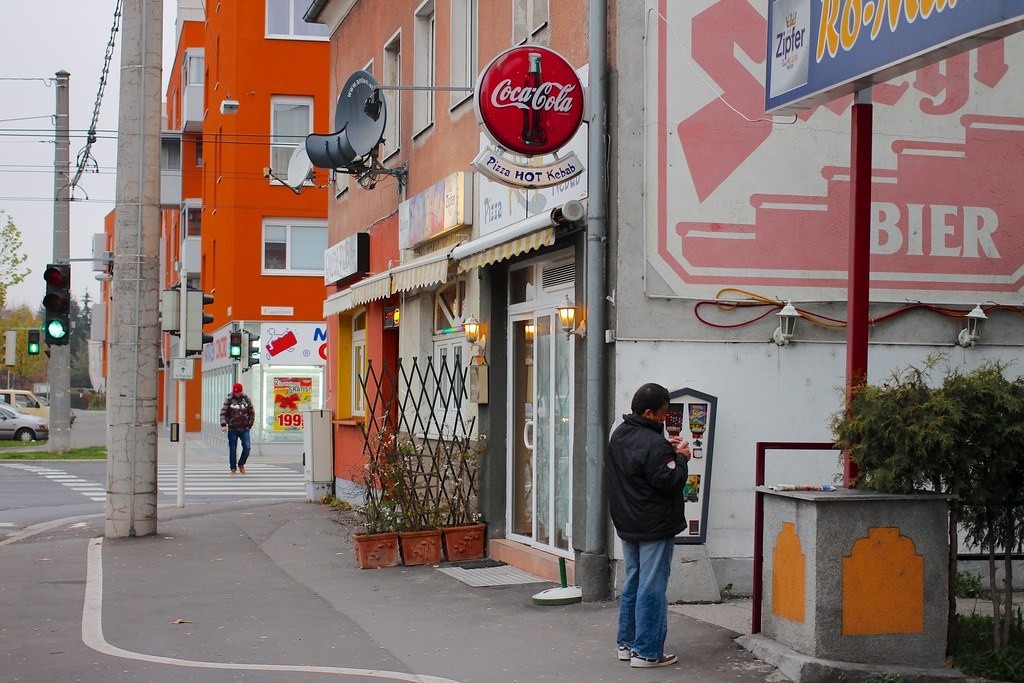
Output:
[323,289,355,318]
[391,238,466,294]
[350,265,391,306]
[451,200,584,275]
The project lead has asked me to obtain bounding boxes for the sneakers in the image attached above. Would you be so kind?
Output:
[230,470,236,475]
[616,643,632,660]
[630,651,678,667]
[239,465,246,474]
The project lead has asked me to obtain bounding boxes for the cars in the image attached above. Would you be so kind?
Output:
[0,388,77,428]
[0,400,50,441]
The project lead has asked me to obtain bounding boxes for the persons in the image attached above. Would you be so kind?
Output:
[604,382,692,668]
[220,383,255,476]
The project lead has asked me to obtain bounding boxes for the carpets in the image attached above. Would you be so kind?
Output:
[435,558,552,586]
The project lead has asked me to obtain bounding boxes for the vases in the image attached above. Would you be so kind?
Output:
[351,521,486,569]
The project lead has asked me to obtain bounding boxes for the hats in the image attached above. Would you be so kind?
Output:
[233,383,243,392]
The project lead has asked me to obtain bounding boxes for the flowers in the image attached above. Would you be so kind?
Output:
[335,423,489,539]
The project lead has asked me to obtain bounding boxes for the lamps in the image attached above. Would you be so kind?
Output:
[555,294,586,340]
[958,303,989,350]
[219,100,240,116]
[462,313,486,350]
[525,323,540,344]
[773,298,801,349]
[363,85,474,122]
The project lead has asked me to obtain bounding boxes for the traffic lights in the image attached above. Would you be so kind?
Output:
[27,329,40,355]
[241,333,260,373]
[2,331,16,367]
[41,322,51,358]
[230,332,241,360]
[185,290,214,356]
[42,263,71,346]
